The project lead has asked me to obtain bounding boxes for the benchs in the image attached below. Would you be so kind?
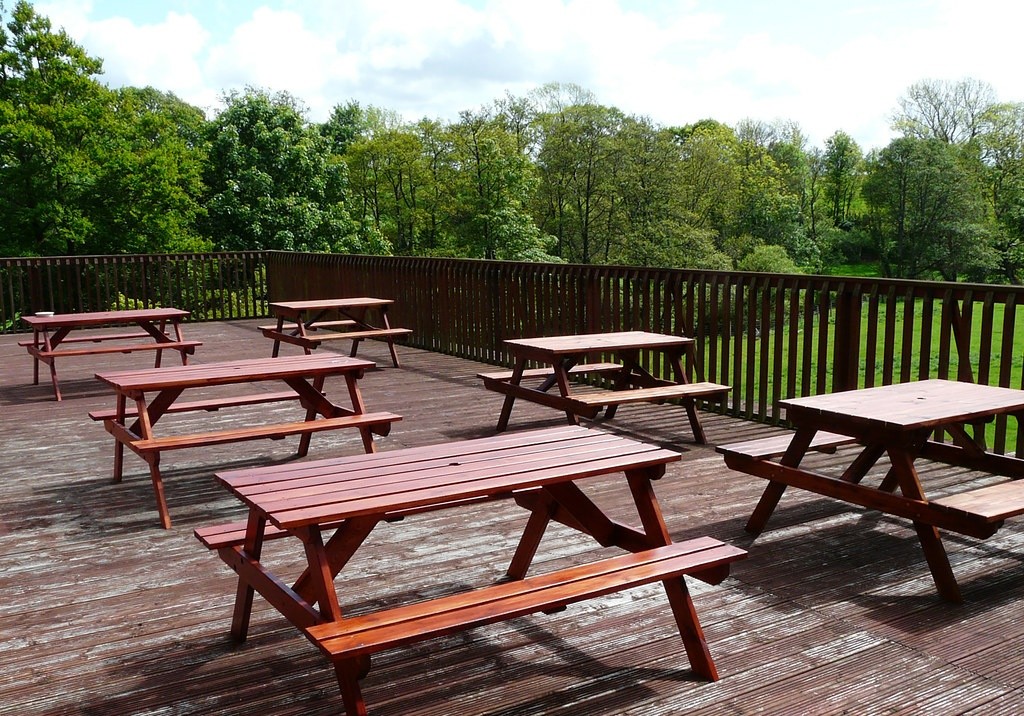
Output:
[566,381,733,406]
[18,330,177,347]
[122,410,404,451]
[86,388,327,420]
[303,328,414,343]
[258,320,367,333]
[307,534,749,657]
[194,487,553,552]
[716,431,855,473]
[37,341,203,358]
[474,362,631,390]
[928,479,1024,519]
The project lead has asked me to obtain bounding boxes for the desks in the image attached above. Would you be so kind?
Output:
[95,353,378,531]
[498,331,707,449]
[739,379,1024,603]
[269,298,400,369]
[219,426,720,716]
[22,307,191,401]
